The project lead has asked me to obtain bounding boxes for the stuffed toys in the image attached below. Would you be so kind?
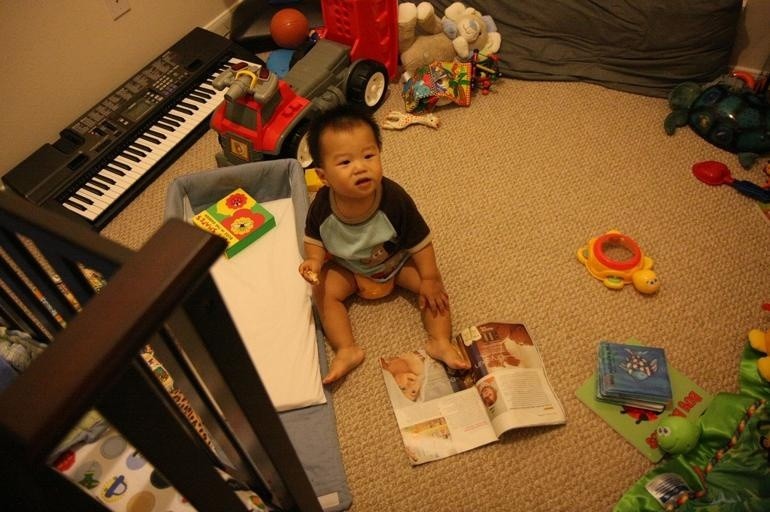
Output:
[393,2,501,80]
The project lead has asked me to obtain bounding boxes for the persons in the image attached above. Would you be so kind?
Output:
[479,381,498,407]
[382,352,428,406]
[477,323,533,367]
[298,105,472,385]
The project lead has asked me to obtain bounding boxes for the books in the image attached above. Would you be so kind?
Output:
[382,322,565,466]
[594,341,671,412]
[192,186,276,257]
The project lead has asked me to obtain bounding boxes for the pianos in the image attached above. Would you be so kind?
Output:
[2,25,273,233]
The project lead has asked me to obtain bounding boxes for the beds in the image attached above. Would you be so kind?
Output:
[2,182,321,511]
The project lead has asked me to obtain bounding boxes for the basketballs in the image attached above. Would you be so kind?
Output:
[270,9,309,49]
